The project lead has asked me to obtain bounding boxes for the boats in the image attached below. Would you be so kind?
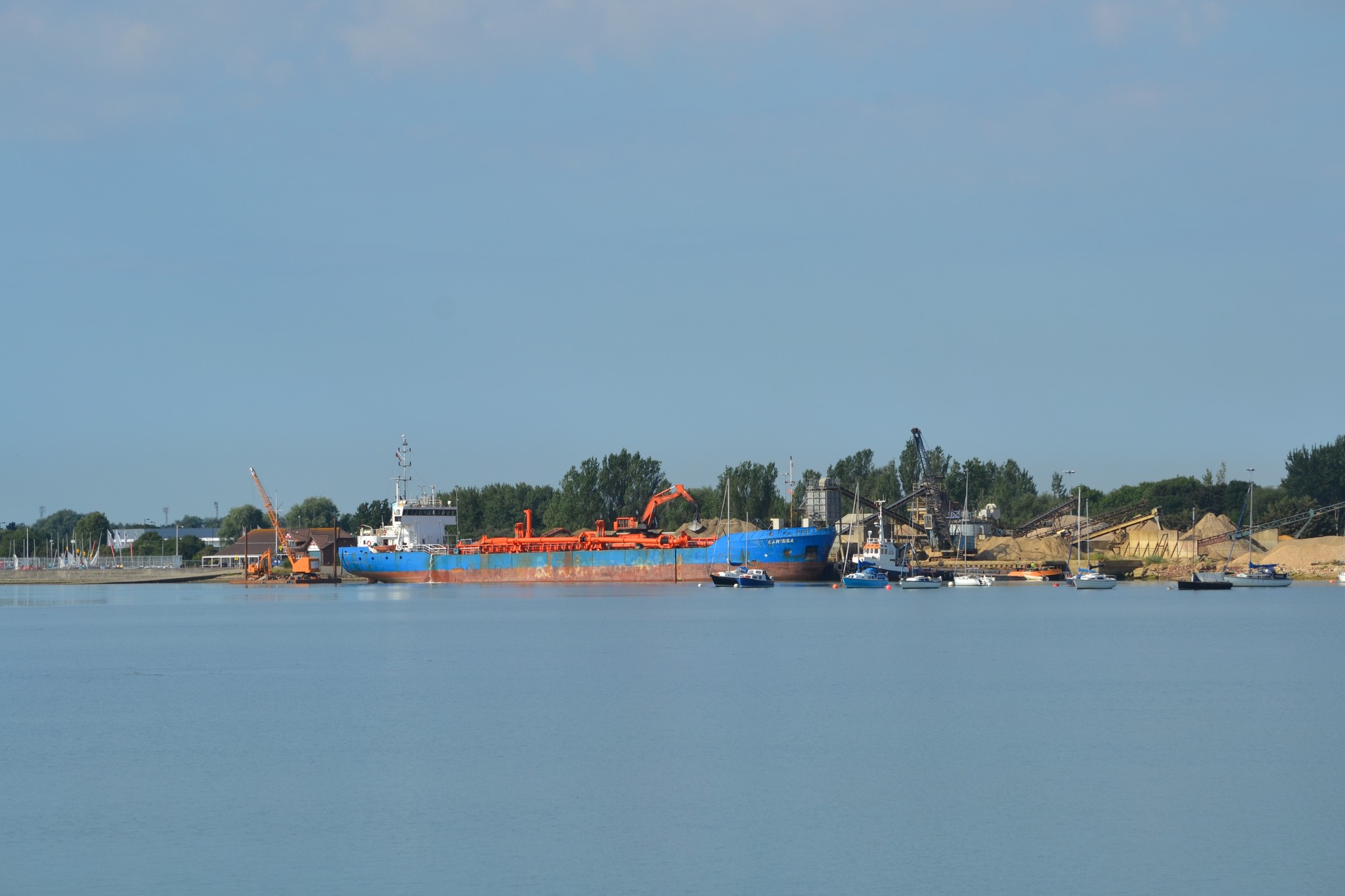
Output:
[736,569,775,589]
[837,493,1064,583]
[337,434,837,585]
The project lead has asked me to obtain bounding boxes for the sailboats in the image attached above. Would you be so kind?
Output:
[1177,506,1233,591]
[952,470,996,587]
[1,527,138,570]
[899,498,942,589]
[1063,487,1100,586]
[1072,498,1118,589]
[708,476,751,586]
[1223,483,1294,587]
[842,479,889,589]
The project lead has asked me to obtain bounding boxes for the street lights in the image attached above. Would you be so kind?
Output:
[1063,470,1076,515]
[1245,468,1255,540]
[789,455,796,528]
[780,472,790,528]
[176,525,184,557]
